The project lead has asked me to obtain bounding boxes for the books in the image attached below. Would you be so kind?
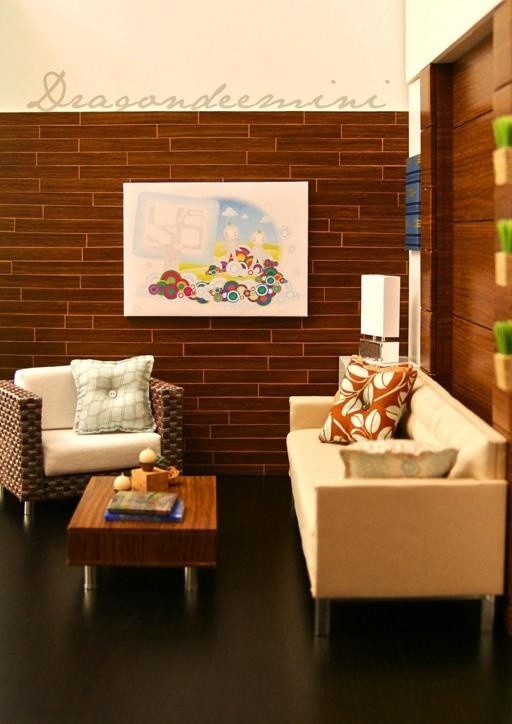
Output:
[107,489,177,514]
[104,499,187,522]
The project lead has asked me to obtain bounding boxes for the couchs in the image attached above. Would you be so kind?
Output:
[285,360,508,639]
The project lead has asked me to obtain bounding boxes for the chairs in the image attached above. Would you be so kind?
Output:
[0,364,186,518]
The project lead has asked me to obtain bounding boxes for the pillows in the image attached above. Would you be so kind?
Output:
[70,353,159,436]
[314,353,462,481]
